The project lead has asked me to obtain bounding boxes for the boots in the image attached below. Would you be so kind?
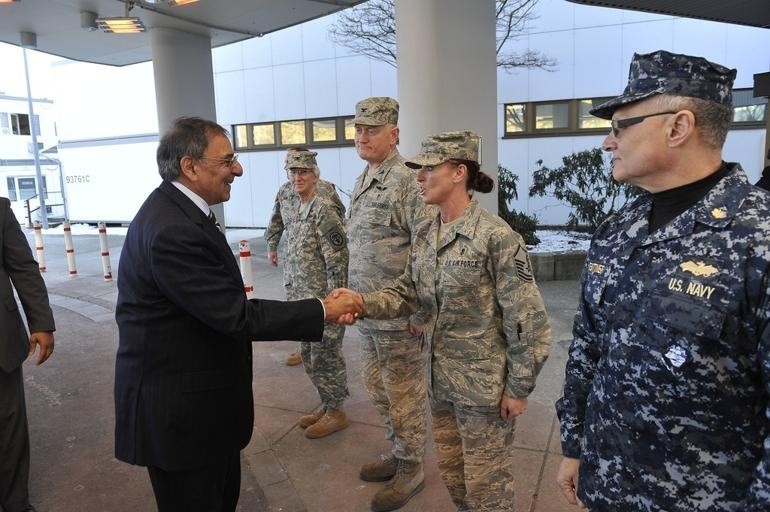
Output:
[371,460,425,511]
[300,409,326,428]
[359,454,398,482]
[305,409,348,439]
[286,352,302,365]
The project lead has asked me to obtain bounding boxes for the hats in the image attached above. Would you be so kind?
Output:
[589,50,737,119]
[286,151,317,169]
[406,131,479,170]
[354,97,400,126]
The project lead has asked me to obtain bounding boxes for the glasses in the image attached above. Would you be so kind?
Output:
[611,111,678,137]
[202,154,238,166]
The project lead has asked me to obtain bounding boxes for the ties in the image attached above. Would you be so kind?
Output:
[208,209,221,233]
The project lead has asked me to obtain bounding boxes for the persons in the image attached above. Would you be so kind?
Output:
[346,96,438,510]
[0,196,55,512]
[111,117,362,510]
[284,151,346,437]
[554,53,770,510]
[263,148,345,367]
[333,132,548,511]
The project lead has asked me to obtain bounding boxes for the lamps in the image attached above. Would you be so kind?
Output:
[163,0,200,9]
[96,1,145,34]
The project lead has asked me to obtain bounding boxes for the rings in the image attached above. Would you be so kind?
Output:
[351,312,360,320]
